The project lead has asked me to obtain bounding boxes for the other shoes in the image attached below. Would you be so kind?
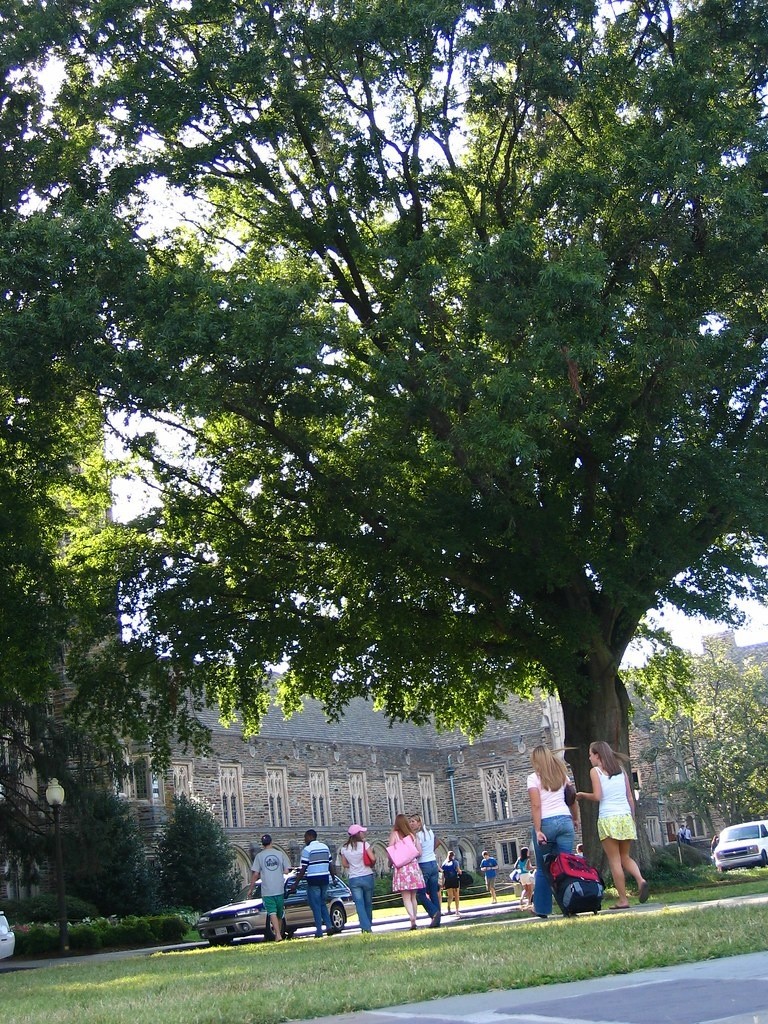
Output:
[430,912,442,928]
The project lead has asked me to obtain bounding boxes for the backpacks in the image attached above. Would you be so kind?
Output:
[442,859,459,878]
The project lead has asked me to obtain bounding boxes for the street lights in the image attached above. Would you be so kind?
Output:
[45,777,65,957]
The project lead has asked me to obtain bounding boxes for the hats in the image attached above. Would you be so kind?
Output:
[347,825,367,836]
[262,835,271,844]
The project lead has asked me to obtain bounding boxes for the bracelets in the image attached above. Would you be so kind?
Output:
[490,866,492,869]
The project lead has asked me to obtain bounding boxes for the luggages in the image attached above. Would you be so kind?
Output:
[538,840,604,918]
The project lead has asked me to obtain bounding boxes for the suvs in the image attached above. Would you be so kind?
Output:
[714,819,768,872]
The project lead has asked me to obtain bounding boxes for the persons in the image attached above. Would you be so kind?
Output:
[526,743,579,919]
[514,847,537,909]
[677,824,693,847]
[711,835,724,874]
[291,829,337,938]
[575,741,651,910]
[480,851,499,904]
[410,814,442,928]
[426,851,462,915]
[387,814,426,930]
[574,843,583,858]
[248,834,292,942]
[341,824,377,934]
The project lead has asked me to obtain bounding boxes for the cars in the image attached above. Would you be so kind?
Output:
[0,911,16,962]
[197,868,357,946]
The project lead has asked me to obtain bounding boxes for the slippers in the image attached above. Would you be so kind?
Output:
[639,882,650,903]
[608,903,630,909]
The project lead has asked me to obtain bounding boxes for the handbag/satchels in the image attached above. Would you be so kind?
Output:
[363,841,372,866]
[386,834,420,869]
[564,783,576,805]
[438,871,445,886]
